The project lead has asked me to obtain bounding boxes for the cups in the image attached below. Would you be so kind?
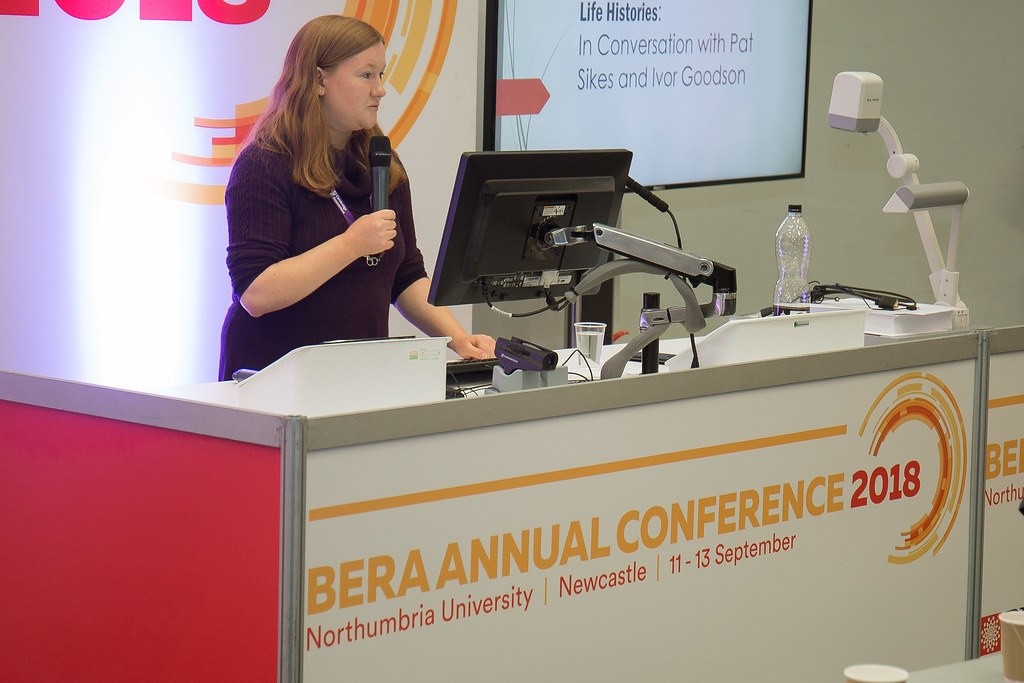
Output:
[998,611,1024,683]
[574,322,608,366]
[842,664,908,683]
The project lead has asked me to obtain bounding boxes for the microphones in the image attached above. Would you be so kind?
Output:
[368,136,392,213]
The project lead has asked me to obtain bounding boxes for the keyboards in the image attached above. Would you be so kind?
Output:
[446,357,501,375]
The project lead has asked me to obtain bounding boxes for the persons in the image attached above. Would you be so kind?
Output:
[218,15,496,382]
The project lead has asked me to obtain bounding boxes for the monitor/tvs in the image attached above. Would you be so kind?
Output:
[427,148,633,307]
[473,0,813,192]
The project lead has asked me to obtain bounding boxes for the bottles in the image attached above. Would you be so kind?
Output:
[772,203,812,306]
